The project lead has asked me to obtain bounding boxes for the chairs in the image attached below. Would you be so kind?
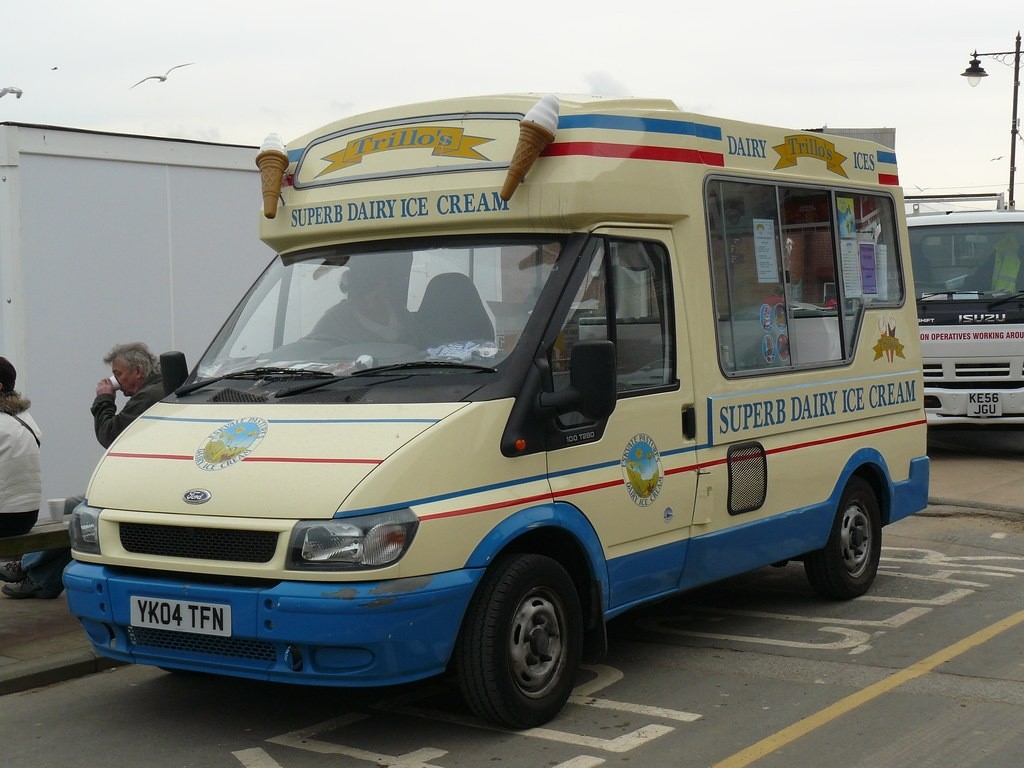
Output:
[418,272,494,348]
[928,266,976,292]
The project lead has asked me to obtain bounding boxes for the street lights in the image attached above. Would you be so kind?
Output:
[960,29,1024,211]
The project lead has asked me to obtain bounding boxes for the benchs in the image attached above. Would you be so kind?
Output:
[0,516,75,563]
[578,307,859,377]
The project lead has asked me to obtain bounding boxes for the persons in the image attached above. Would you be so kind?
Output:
[259,264,419,368]
[0,356,42,539]
[0,343,166,599]
[991,231,1021,293]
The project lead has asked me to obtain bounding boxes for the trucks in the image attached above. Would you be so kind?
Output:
[63,93,930,730]
[901,193,1024,424]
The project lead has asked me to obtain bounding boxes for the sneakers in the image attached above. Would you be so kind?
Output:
[0,560,28,583]
[2,583,57,599]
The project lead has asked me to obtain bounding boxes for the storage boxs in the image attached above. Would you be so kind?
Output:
[486,300,599,372]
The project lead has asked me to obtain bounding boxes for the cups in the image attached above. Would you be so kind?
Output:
[110,375,121,390]
[47,498,66,521]
